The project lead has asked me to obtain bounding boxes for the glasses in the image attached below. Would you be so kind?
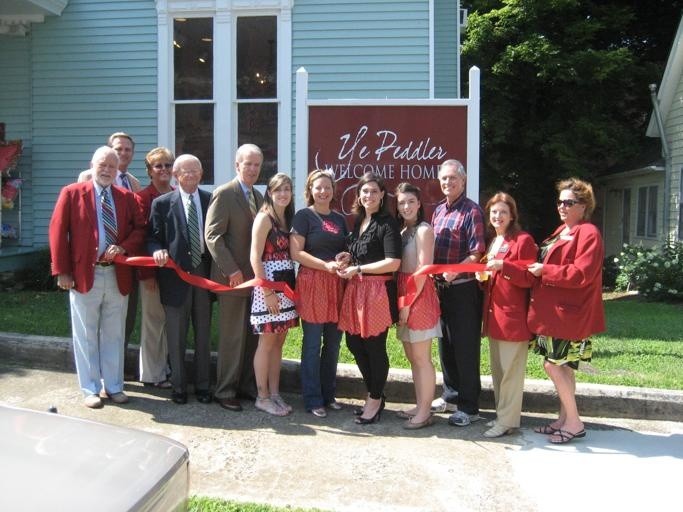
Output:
[155,164,172,168]
[556,199,580,207]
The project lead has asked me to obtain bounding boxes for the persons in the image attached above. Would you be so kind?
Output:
[248,172,295,417]
[334,171,402,425]
[76,131,141,382]
[147,152,212,406]
[429,158,486,428]
[204,143,265,413]
[526,176,605,445]
[48,145,145,410]
[136,146,174,390]
[287,168,349,420]
[476,191,539,438]
[394,182,442,430]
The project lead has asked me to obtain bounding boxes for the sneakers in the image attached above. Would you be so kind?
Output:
[84,392,128,407]
[431,397,479,426]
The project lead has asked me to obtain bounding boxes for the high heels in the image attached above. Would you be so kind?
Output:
[352,394,385,424]
[483,419,514,438]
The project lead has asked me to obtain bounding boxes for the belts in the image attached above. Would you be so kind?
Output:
[95,262,113,266]
[437,277,475,286]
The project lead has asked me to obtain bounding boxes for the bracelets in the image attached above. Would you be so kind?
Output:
[356,265,361,274]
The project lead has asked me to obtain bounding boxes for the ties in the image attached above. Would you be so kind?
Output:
[248,191,257,221]
[187,195,201,269]
[120,174,129,190]
[102,190,118,252]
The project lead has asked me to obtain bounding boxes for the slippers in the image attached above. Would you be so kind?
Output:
[534,423,586,444]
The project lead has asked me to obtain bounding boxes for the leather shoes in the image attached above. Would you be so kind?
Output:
[154,381,257,411]
[395,409,434,429]
[311,402,341,418]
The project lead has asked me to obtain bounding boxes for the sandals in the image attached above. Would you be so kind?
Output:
[254,394,293,416]
